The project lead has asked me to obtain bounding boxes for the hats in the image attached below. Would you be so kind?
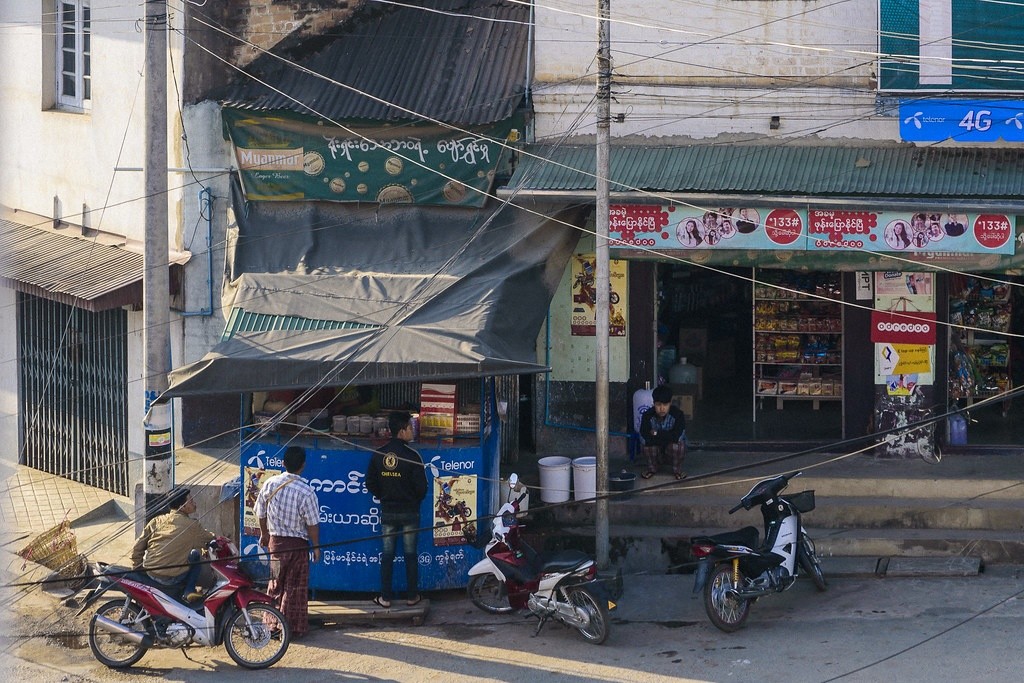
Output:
[652,385,673,400]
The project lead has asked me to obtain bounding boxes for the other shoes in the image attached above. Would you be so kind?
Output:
[187,593,208,604]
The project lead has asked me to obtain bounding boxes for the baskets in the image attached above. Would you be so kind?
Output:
[781,490,816,513]
[17,519,87,592]
[238,559,280,582]
[462,514,494,549]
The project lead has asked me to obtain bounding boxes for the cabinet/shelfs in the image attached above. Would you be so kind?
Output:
[752,265,845,440]
[946,274,1013,417]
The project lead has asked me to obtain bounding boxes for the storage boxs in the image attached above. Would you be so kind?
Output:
[670,383,699,421]
[679,324,708,358]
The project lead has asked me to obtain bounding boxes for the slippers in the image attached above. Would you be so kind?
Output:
[641,469,658,479]
[407,594,423,606]
[672,470,686,480]
[373,595,392,608]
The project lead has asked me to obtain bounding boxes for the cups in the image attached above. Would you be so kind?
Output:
[297,408,387,438]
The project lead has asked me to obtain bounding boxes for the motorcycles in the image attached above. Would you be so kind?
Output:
[689,471,827,633]
[467,473,612,646]
[73,533,291,670]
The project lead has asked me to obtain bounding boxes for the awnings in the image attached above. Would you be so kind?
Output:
[153,171,593,401]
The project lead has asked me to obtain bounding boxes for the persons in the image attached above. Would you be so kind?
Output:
[639,385,687,480]
[365,412,429,609]
[253,446,322,642]
[129,488,216,637]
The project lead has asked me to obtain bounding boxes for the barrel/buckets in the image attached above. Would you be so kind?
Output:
[572,456,596,504]
[538,456,572,503]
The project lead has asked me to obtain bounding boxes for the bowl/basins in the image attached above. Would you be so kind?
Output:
[610,472,637,501]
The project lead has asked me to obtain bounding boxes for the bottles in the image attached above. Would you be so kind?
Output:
[670,357,697,394]
